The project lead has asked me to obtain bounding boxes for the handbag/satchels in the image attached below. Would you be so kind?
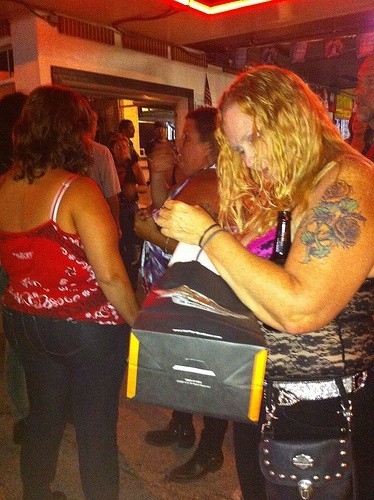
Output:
[258,438,357,500]
[126,229,267,424]
[138,246,169,295]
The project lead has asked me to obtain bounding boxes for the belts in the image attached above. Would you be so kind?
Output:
[261,371,368,406]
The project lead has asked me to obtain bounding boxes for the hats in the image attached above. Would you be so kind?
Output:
[154,121,168,129]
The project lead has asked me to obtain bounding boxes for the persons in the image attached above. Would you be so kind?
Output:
[134,105,229,483]
[82,56,374,294]
[155,66,374,500]
[0,84,139,500]
[1,90,30,447]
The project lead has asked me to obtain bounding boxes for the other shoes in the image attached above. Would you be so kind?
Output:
[130,271,138,291]
[13,417,27,444]
[0,397,12,414]
[51,491,68,500]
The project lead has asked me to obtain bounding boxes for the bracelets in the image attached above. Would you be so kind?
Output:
[198,221,220,246]
[163,235,169,255]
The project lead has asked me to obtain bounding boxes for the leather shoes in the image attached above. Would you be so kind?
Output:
[145,421,197,448]
[167,450,225,482]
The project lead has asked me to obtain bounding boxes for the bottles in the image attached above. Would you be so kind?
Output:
[263,211,293,332]
